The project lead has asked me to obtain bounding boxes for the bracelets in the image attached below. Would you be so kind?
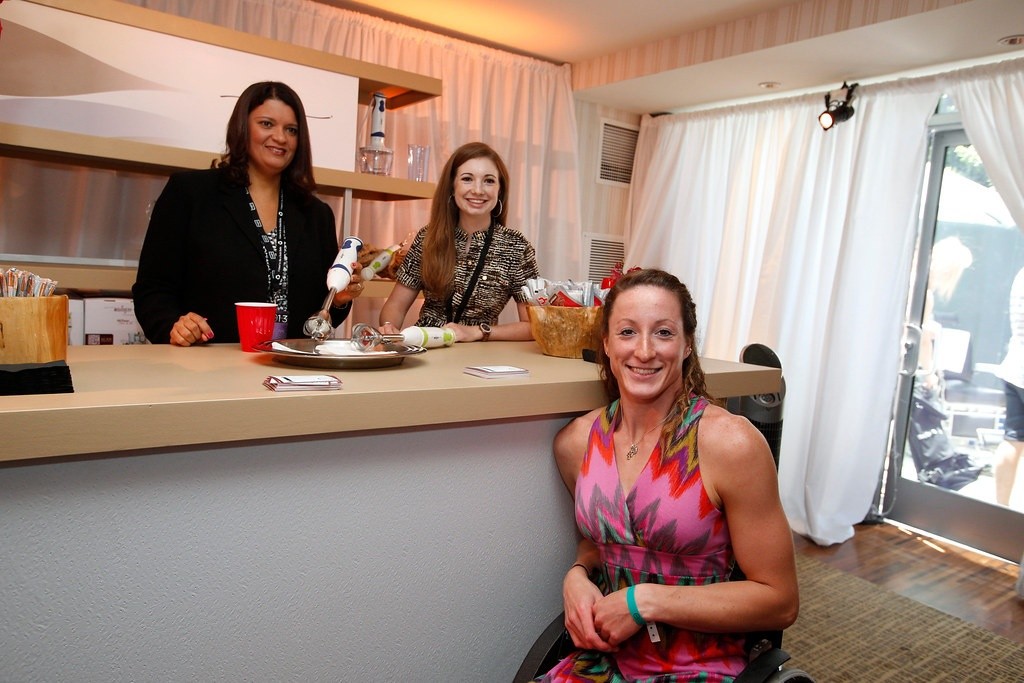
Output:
[626,585,647,626]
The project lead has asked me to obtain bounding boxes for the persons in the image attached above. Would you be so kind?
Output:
[132,81,365,347]
[375,142,541,342]
[908,237,1024,506]
[526,268,798,683]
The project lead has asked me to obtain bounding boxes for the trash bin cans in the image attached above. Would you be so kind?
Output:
[725,344,786,474]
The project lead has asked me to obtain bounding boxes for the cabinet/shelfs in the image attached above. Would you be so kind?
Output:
[0,0,442,203]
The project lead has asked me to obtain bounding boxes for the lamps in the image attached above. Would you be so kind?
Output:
[818,81,862,132]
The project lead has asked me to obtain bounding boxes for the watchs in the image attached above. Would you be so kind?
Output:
[479,323,491,341]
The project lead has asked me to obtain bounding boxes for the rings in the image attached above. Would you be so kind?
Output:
[385,322,391,324]
[358,284,361,287]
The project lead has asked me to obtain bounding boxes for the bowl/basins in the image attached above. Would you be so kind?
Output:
[526,305,608,359]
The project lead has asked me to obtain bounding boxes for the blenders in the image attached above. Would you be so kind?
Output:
[359,91,394,177]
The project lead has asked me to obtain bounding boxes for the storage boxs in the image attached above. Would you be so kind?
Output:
[68,295,151,345]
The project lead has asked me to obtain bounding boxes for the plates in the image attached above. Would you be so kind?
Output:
[251,339,428,369]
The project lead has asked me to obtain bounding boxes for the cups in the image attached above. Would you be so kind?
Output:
[407,144,430,183]
[234,302,278,352]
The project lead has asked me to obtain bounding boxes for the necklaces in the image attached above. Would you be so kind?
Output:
[619,399,673,460]
